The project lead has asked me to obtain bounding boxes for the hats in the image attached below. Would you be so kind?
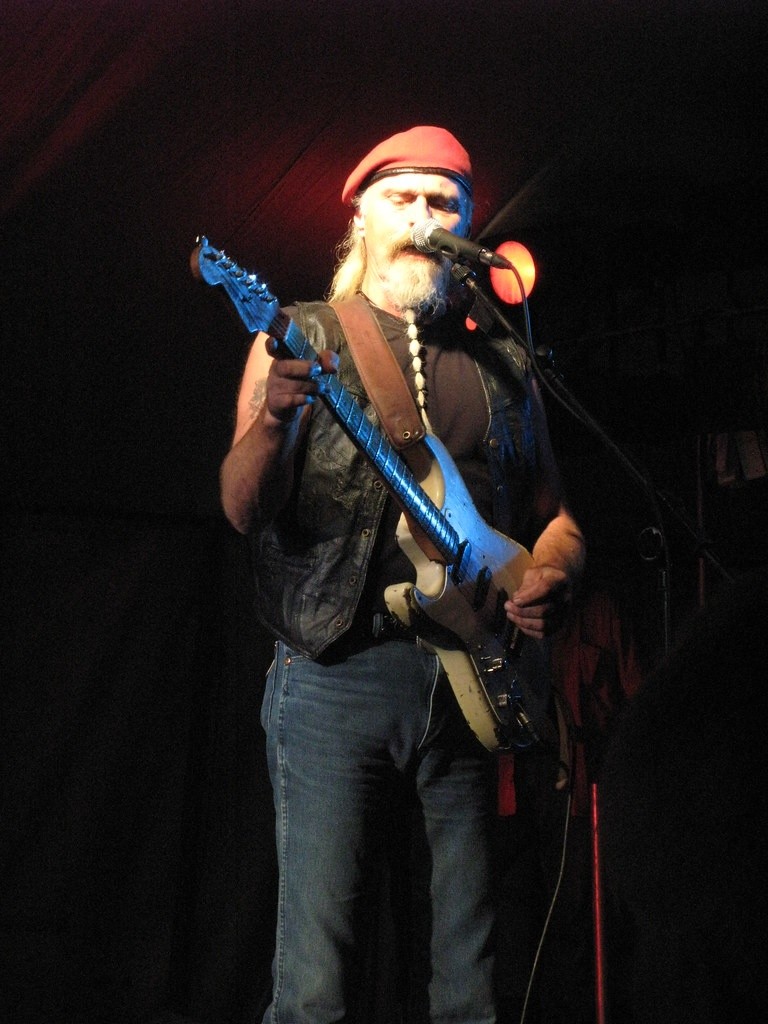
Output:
[341,126,474,207]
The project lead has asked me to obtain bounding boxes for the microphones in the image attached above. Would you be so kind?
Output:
[409,218,511,270]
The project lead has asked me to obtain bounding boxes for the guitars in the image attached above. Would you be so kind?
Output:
[188,232,552,756]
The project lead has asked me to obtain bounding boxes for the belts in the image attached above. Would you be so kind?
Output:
[350,613,418,644]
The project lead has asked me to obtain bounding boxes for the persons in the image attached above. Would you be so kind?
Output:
[492,578,654,927]
[220,125,588,1024]
